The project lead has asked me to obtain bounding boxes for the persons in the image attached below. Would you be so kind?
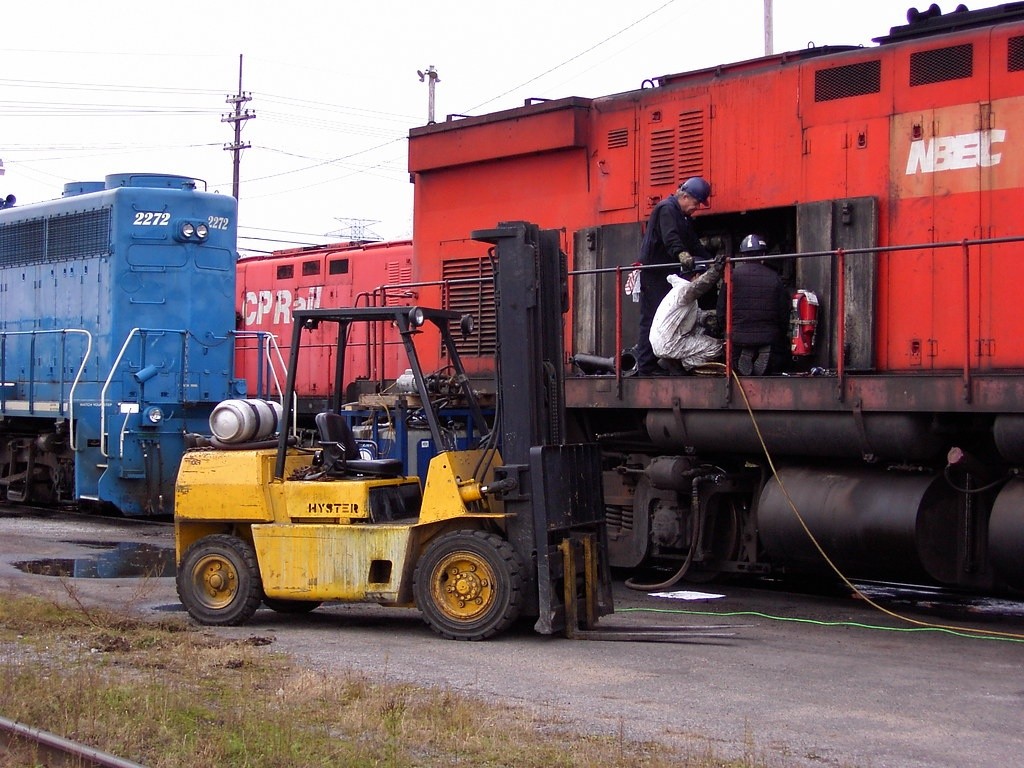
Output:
[648,254,729,376]
[636,177,712,375]
[716,235,791,375]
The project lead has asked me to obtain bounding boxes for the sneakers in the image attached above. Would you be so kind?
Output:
[658,358,685,374]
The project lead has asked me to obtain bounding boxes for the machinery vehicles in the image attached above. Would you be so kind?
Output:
[158,220,617,642]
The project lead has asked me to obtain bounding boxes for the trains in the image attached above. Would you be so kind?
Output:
[0,172,417,526]
[407,0,1024,614]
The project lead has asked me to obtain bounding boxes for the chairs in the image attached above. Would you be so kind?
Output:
[313,412,403,479]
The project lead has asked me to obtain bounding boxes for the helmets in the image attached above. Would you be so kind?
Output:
[739,234,767,253]
[679,177,710,206]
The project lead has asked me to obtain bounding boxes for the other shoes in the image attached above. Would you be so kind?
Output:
[738,345,773,375]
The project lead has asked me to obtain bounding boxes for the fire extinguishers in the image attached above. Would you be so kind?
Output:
[789,288,819,365]
